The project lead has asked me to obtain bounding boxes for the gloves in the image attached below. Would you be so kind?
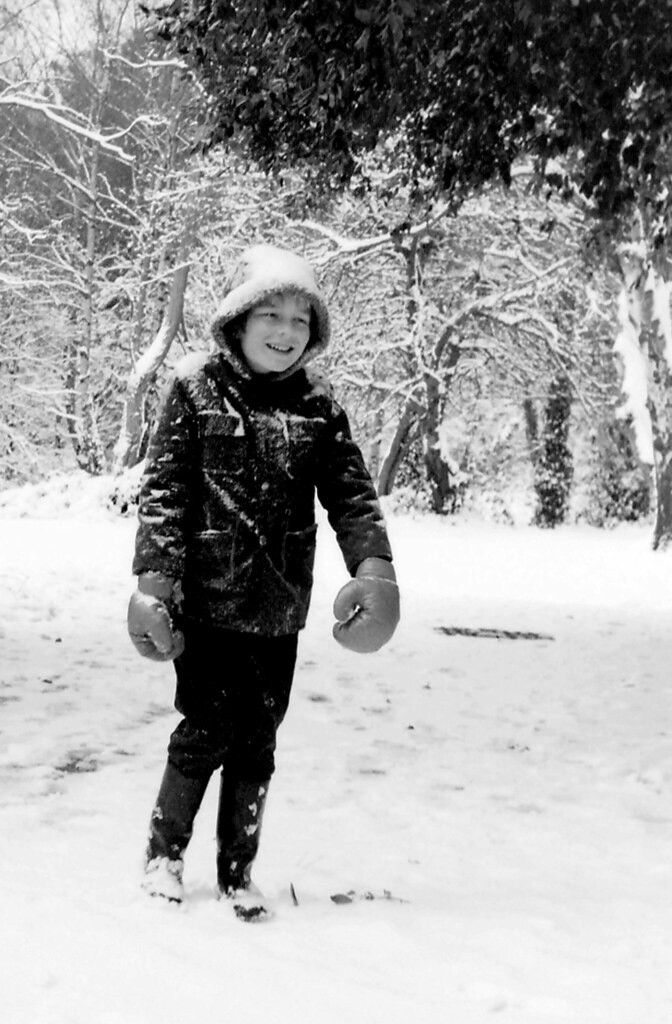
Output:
[333,556,400,654]
[127,571,185,662]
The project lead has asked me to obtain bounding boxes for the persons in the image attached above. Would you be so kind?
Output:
[126,245,403,923]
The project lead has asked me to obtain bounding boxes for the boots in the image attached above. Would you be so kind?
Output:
[217,770,273,922]
[140,762,211,905]
